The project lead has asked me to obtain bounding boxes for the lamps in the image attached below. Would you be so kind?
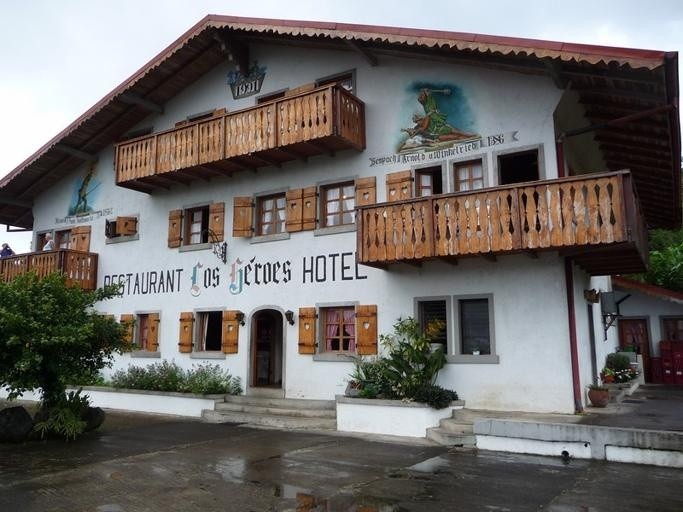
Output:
[235,310,244,326]
[600,291,623,342]
[284,309,294,326]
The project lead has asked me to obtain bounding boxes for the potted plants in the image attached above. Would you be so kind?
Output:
[471,340,480,354]
[588,383,608,407]
[422,318,446,356]
[601,367,615,384]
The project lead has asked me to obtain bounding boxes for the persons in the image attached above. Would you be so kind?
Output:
[42,232,56,250]
[0,243,15,256]
[404,87,476,142]
